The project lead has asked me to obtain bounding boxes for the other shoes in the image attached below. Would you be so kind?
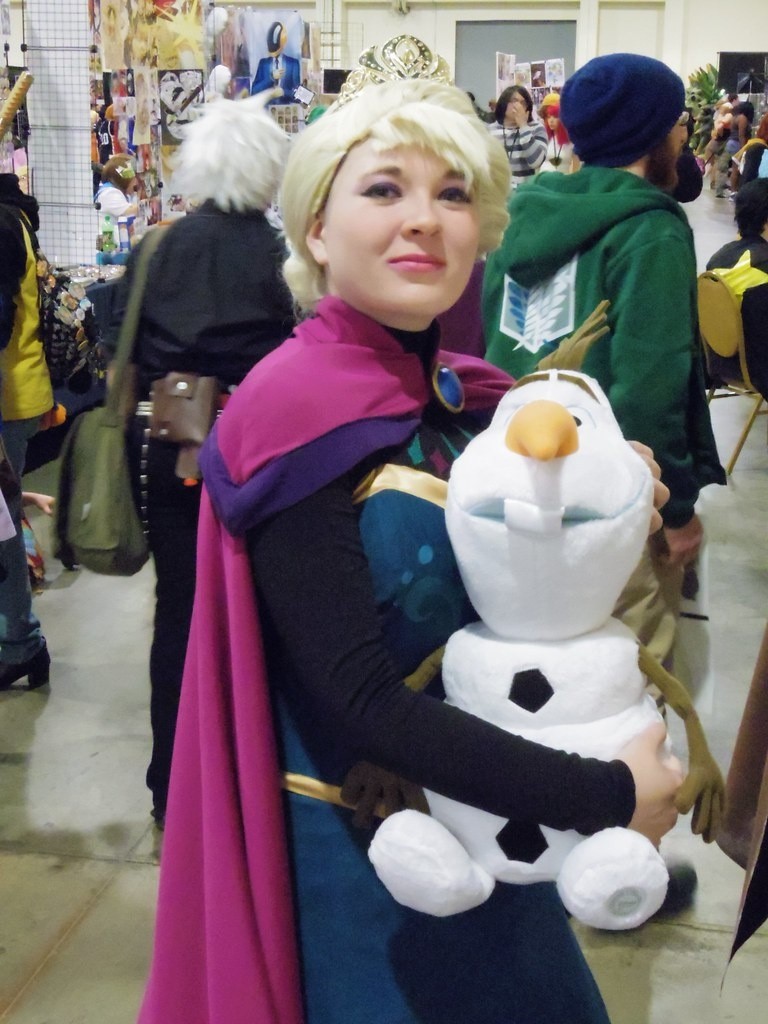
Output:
[593,865,699,935]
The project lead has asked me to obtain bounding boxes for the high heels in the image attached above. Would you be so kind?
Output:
[0,646,51,693]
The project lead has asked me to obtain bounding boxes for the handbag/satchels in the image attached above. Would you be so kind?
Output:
[50,408,151,579]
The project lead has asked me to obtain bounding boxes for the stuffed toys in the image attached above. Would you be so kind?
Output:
[342,300,726,932]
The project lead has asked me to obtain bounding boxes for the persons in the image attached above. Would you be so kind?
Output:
[465,92,487,123]
[132,75,619,1021]
[477,53,729,734]
[0,170,77,691]
[726,101,755,202]
[487,85,548,190]
[680,90,739,198]
[697,178,768,403]
[537,93,581,175]
[54,99,301,865]
[22,492,56,518]
[436,258,486,361]
[251,21,302,104]
[731,112,768,185]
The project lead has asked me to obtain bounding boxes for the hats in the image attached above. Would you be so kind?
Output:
[560,53,685,168]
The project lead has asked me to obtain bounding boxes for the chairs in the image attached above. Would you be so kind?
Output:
[686,270,768,477]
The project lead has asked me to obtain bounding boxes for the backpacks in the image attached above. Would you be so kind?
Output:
[1,201,96,365]
